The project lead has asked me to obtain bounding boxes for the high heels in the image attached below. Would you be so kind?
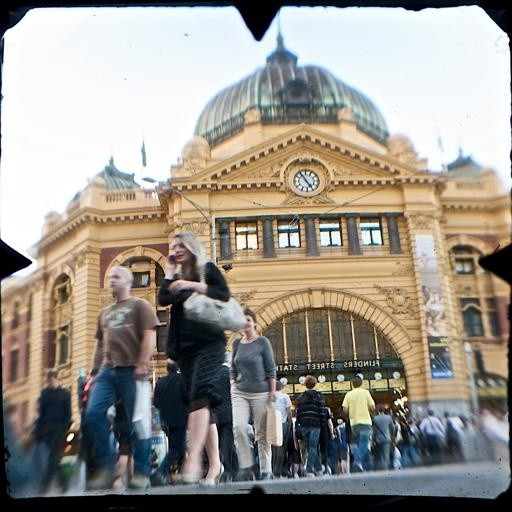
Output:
[199,464,225,488]
[178,461,203,484]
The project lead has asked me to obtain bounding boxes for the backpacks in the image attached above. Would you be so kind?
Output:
[295,420,304,439]
[447,428,458,444]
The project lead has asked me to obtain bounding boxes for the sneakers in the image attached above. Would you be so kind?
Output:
[303,462,365,477]
[87,468,170,491]
[235,470,253,481]
[260,471,301,482]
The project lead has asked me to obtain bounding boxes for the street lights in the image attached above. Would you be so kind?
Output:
[143,177,217,263]
[463,337,479,413]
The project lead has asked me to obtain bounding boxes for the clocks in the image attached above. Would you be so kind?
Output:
[288,164,327,199]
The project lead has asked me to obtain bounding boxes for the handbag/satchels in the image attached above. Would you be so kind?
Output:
[131,376,153,440]
[184,260,246,332]
[435,433,447,449]
[265,400,284,447]
[371,442,376,456]
